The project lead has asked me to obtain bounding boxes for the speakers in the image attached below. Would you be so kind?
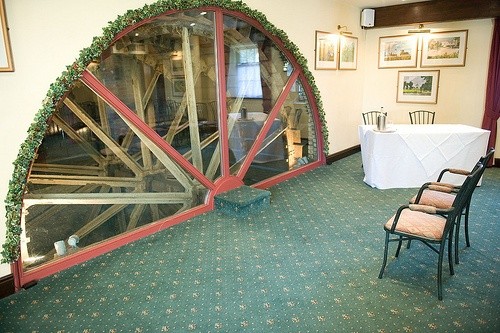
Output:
[361,9,375,28]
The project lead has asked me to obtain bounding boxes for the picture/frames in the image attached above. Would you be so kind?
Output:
[170,56,185,97]
[378,34,419,69]
[315,30,358,70]
[0,0,15,73]
[420,29,469,68]
[396,69,440,104]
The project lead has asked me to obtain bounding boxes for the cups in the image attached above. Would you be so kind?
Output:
[53,240,68,256]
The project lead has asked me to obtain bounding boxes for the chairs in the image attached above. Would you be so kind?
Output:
[407,147,496,263]
[409,110,436,124]
[280,106,302,135]
[378,162,484,301]
[361,111,387,168]
[167,100,217,147]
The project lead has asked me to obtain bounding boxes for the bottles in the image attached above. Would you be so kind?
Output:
[241,108,247,119]
[377,106,385,132]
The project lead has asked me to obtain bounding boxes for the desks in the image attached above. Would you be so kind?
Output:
[359,124,492,190]
[227,111,286,164]
